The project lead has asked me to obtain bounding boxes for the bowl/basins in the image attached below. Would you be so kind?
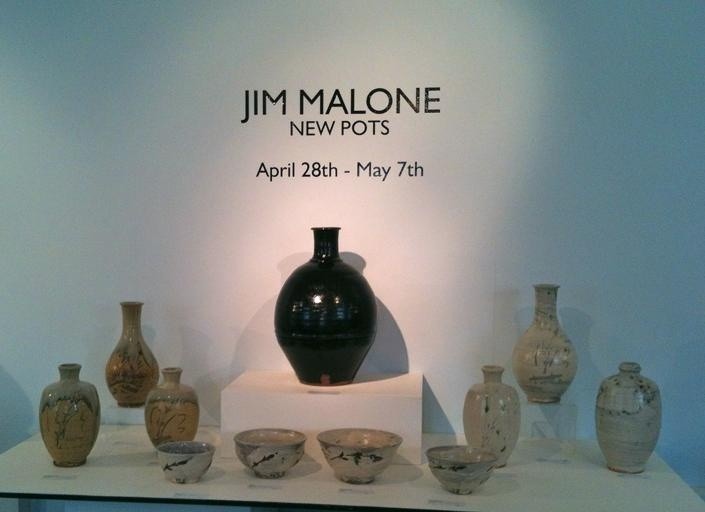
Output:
[316,425,403,485]
[158,440,215,485]
[234,426,309,478]
[425,445,499,497]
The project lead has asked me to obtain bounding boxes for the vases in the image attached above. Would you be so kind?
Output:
[35,299,202,469]
[462,282,663,476]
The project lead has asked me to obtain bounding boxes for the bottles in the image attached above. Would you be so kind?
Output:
[39,300,198,468]
[464,284,663,474]
